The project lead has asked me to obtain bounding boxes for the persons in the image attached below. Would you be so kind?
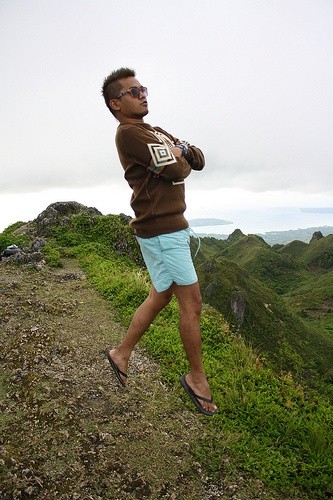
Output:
[101,68,218,416]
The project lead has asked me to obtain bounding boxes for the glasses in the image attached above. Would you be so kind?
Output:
[116,87,148,99]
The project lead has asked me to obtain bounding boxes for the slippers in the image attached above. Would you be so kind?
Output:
[180,374,217,417]
[107,351,128,387]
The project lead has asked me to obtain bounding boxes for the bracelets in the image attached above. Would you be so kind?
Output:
[177,143,189,157]
